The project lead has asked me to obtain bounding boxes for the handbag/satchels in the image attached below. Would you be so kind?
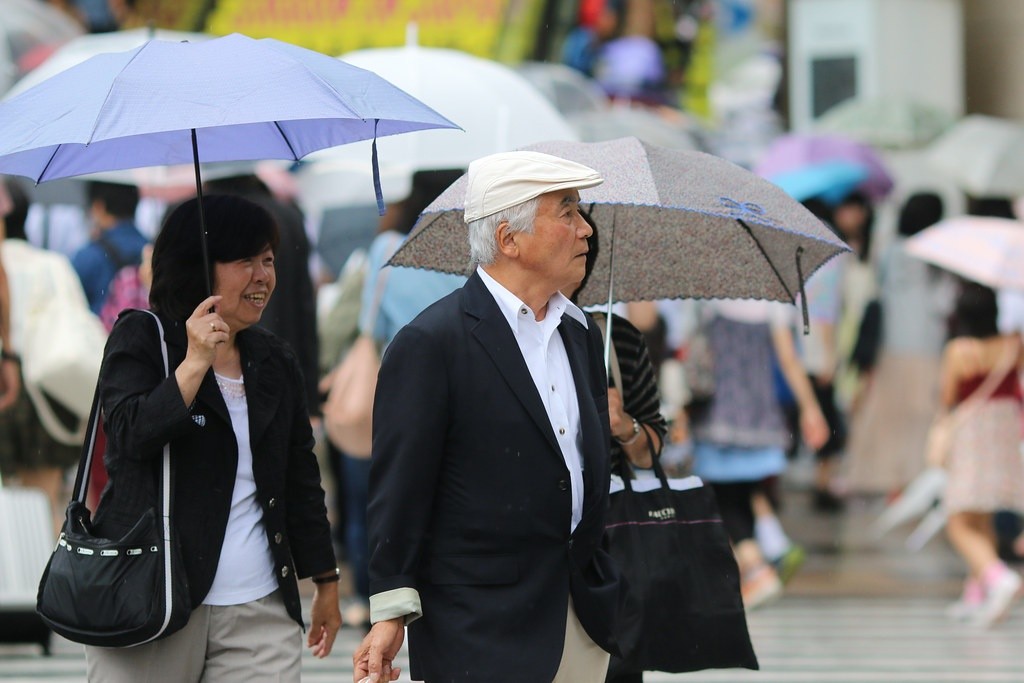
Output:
[35,502,188,647]
[673,300,717,397]
[323,338,381,459]
[570,418,759,672]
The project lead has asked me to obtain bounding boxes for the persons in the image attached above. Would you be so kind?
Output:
[0,171,1024,683]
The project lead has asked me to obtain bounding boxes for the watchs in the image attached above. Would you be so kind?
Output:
[309,566,342,584]
[615,418,640,446]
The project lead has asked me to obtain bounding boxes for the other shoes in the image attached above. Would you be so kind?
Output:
[741,566,779,607]
[769,544,808,585]
[971,575,1020,629]
[943,577,983,617]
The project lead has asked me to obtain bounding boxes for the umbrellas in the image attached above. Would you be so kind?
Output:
[0,5,1024,388]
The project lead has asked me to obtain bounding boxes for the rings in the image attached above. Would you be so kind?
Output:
[209,322,216,332]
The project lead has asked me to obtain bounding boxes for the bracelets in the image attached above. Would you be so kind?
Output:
[2,347,17,361]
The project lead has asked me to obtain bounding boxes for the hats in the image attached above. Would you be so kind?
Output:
[462,152,607,224]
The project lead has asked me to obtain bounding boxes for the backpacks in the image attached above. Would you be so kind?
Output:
[94,241,150,330]
